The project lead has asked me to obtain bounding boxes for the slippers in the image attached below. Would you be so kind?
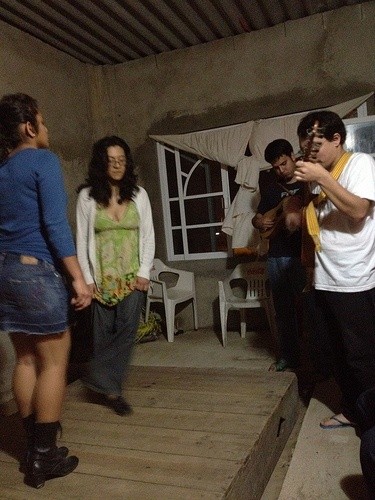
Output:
[320,413,354,428]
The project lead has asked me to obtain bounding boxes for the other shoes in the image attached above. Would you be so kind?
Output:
[271,350,302,372]
[311,362,333,382]
[101,389,133,416]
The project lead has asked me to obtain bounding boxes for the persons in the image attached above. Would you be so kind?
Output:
[76,136,155,411]
[0,93,91,487]
[253,111,375,500]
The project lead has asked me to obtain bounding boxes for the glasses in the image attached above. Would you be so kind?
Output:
[107,159,127,166]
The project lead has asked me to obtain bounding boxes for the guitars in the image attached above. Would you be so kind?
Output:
[290,122,327,235]
[254,190,300,241]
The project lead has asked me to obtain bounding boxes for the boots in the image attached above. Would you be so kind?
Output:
[21,411,79,488]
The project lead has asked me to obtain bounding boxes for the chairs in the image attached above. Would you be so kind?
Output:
[218,261,278,351]
[146,259,198,343]
